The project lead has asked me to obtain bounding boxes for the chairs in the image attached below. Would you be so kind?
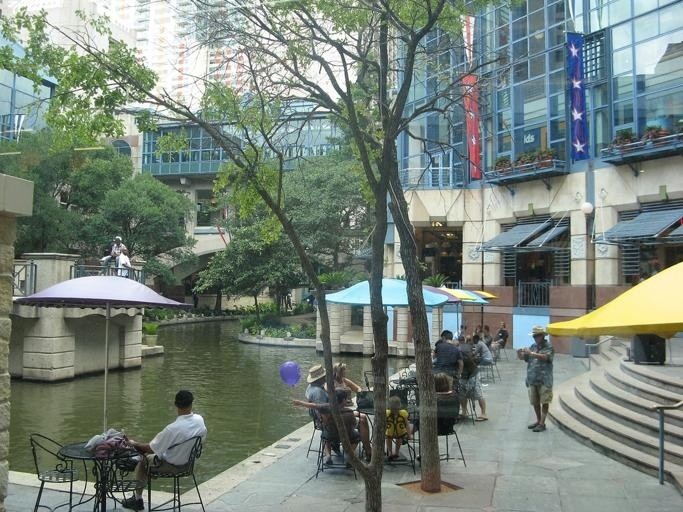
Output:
[143,437,207,512]
[91,448,149,512]
[306,390,467,478]
[361,334,509,425]
[28,431,87,511]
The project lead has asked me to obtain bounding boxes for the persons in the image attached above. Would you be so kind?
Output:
[323,362,362,406]
[384,395,411,461]
[100,236,132,278]
[292,388,360,469]
[433,321,509,434]
[516,325,556,433]
[121,390,208,510]
[304,364,342,456]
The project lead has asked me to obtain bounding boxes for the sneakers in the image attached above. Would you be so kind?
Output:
[122,496,145,511]
[528,424,546,432]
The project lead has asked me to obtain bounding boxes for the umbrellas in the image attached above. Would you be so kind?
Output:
[14,274,194,434]
[325,277,499,318]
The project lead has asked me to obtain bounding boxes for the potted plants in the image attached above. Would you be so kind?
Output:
[144,322,160,348]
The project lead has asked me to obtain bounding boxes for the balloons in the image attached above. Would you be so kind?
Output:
[279,361,301,387]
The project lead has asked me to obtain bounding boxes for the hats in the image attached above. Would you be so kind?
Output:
[529,326,549,337]
[307,365,326,384]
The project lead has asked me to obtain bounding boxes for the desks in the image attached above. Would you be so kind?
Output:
[55,441,152,511]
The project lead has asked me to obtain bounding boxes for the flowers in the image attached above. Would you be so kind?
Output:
[489,147,558,178]
[605,117,682,159]
